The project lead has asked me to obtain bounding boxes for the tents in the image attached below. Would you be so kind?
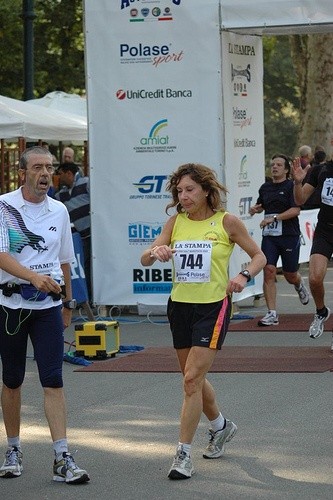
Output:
[0,96,88,189]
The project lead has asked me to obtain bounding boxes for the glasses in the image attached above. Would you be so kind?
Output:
[24,166,55,173]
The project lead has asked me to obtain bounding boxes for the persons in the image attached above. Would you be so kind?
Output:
[0,145,92,484]
[47,145,94,310]
[140,162,267,480]
[248,145,333,339]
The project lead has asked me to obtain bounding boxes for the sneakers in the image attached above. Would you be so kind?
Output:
[258,311,280,325]
[202,418,237,458]
[0,446,23,477]
[52,450,90,483]
[309,307,330,339]
[167,442,195,478]
[295,278,309,305]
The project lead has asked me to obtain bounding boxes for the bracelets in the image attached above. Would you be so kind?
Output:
[150,246,159,260]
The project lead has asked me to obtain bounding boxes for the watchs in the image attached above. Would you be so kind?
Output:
[274,215,277,221]
[62,299,77,310]
[239,269,251,282]
